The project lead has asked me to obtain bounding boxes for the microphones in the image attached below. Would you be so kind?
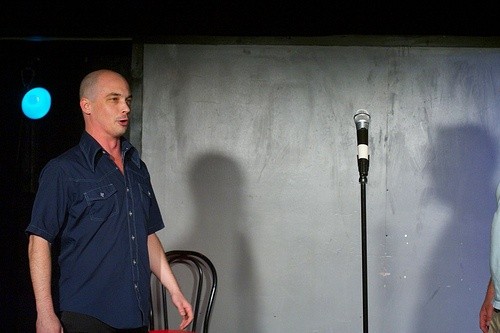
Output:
[354,109,371,181]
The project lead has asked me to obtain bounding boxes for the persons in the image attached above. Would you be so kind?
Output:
[480,183,500,333]
[26,69,196,333]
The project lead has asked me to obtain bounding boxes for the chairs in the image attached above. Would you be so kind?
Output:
[152,250,217,333]
